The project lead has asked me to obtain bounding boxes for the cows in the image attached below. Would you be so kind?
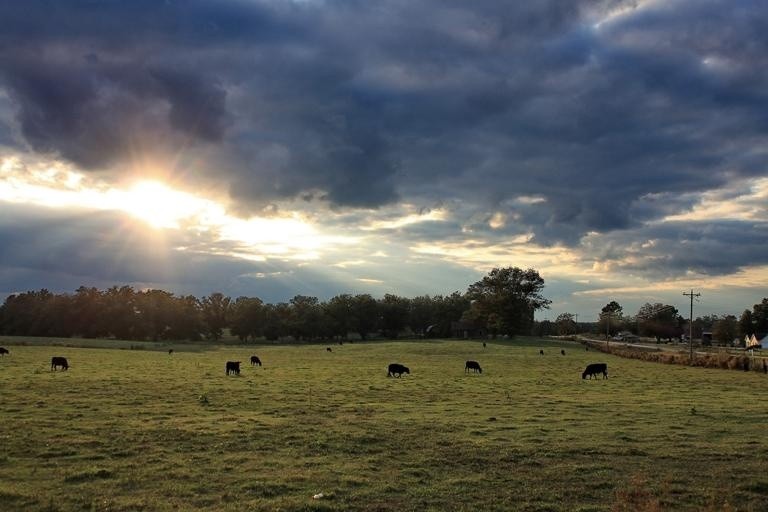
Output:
[465,360,481,374]
[250,355,262,367]
[226,361,242,375]
[388,364,409,378]
[581,363,609,380]
[0,347,9,356]
[51,356,69,371]
